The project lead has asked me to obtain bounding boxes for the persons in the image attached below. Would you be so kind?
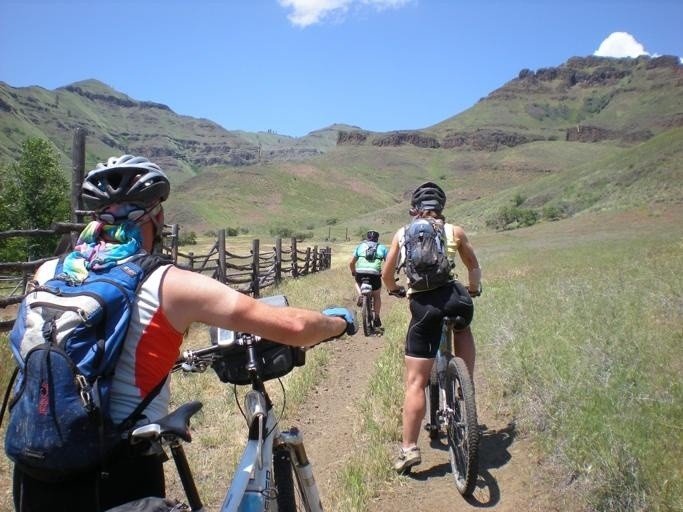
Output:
[349,228,389,327]
[379,183,482,476]
[1,152,359,512]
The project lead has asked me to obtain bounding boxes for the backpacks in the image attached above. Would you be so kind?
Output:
[3,244,176,472]
[401,218,451,284]
[362,240,378,260]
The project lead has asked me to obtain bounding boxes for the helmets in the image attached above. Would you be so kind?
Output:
[79,153,171,209]
[410,181,446,209]
[365,230,379,239]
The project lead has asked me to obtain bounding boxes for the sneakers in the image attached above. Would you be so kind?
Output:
[357,295,364,307]
[393,444,421,474]
[373,318,382,328]
[458,387,464,399]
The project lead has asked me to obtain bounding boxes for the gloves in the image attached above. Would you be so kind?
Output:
[319,302,358,339]
[466,285,482,298]
[387,285,406,299]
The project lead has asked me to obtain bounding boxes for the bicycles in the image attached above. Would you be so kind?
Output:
[108,335,324,512]
[358,277,379,339]
[388,276,479,498]
